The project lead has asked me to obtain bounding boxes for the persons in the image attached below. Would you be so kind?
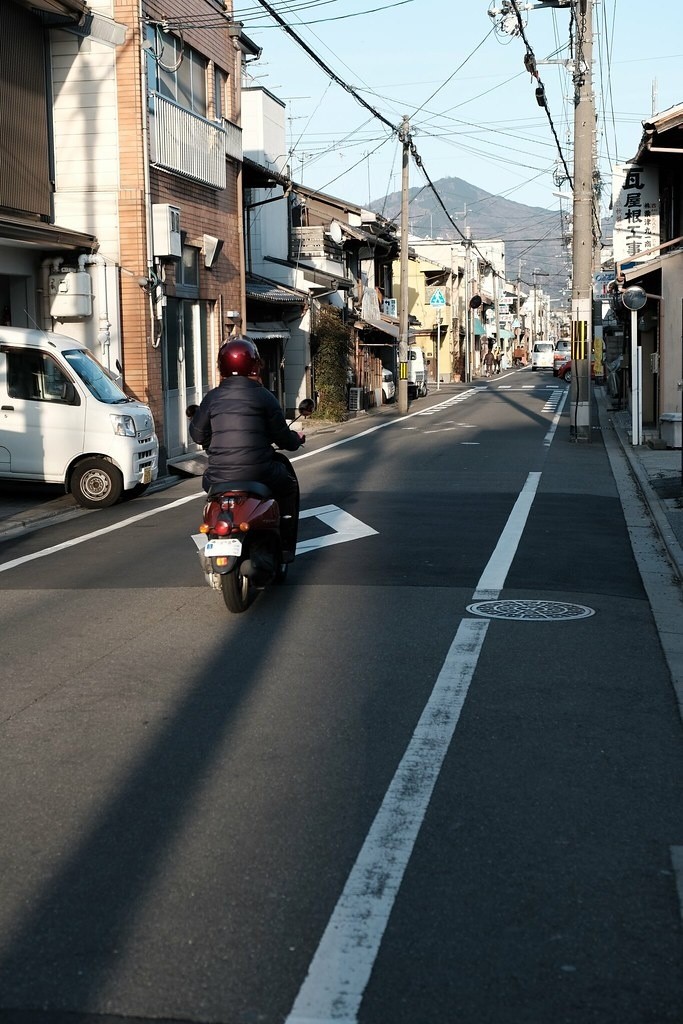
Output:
[492,346,504,375]
[514,346,525,366]
[482,350,496,377]
[504,347,512,371]
[188,334,300,564]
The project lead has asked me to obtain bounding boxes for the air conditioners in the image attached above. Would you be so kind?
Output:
[348,387,364,412]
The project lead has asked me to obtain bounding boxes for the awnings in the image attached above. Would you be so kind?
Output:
[474,318,486,334]
[500,329,515,338]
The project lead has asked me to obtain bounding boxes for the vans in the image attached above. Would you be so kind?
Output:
[0,324,160,510]
[346,351,397,404]
[531,340,556,370]
[552,337,571,378]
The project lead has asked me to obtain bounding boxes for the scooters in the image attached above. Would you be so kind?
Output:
[183,396,319,613]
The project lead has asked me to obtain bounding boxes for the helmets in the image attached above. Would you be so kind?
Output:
[218,339,259,377]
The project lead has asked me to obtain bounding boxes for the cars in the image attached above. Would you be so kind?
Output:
[556,358,606,383]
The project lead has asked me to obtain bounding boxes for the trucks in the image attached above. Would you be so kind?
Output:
[396,347,431,399]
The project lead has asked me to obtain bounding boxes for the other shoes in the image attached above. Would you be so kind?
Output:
[280,550,294,564]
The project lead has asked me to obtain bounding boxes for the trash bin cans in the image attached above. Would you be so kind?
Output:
[659,413,683,449]
